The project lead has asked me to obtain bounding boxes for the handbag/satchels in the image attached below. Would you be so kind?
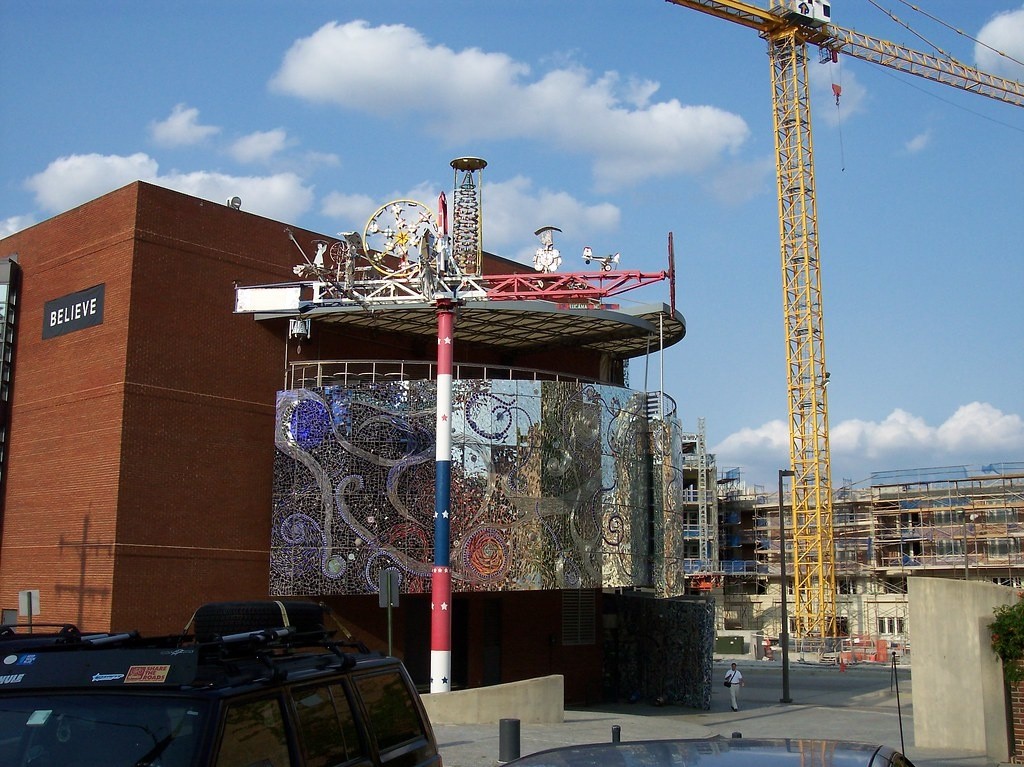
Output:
[724,681,732,688]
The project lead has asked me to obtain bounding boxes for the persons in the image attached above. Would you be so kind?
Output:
[724,663,744,712]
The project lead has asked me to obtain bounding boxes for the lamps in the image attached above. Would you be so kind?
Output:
[226,195,242,210]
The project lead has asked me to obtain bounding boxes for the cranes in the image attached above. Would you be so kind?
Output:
[662,1,1023,651]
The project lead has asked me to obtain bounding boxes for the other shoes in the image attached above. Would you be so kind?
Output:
[731,706,737,712]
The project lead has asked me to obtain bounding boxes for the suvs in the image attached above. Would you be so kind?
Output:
[1,600,444,767]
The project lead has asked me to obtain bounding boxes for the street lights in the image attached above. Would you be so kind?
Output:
[778,469,795,705]
